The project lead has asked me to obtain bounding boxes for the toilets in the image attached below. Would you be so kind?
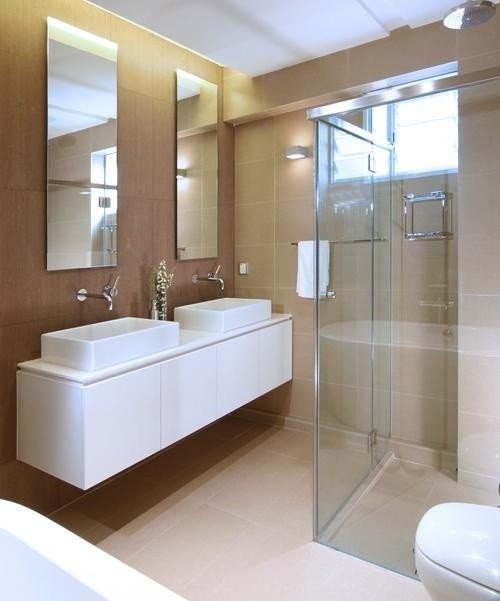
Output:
[415,499,499,599]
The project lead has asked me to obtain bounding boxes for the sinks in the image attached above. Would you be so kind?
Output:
[175,296,274,332]
[39,316,181,373]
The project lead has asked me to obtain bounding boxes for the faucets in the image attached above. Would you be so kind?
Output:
[81,275,121,311]
[193,263,227,291]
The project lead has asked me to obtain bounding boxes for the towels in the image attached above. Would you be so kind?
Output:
[296,241,330,299]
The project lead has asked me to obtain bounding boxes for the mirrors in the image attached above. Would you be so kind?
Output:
[172,66,220,264]
[45,17,125,272]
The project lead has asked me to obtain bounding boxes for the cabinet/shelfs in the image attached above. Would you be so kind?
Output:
[218,317,293,417]
[16,331,218,491]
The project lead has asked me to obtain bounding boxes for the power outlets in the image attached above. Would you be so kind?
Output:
[238,262,248,276]
